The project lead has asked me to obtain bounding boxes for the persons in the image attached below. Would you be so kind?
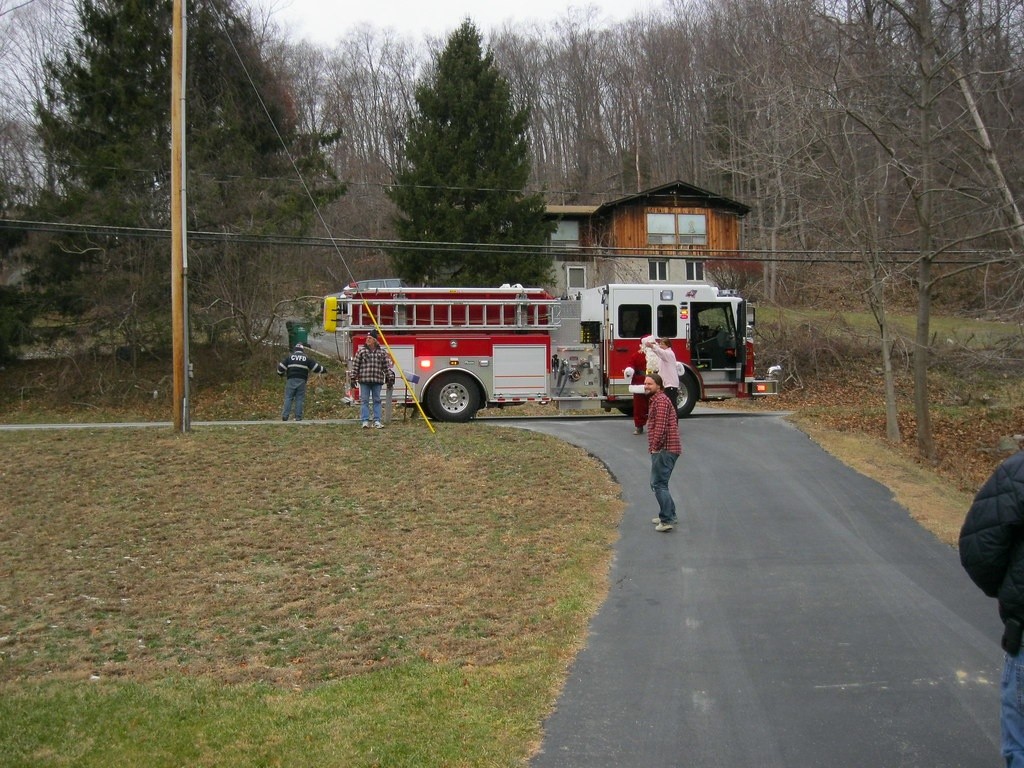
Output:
[350,329,395,429]
[623,335,661,435]
[644,374,681,531]
[959,449,1024,768]
[277,342,327,421]
[646,338,679,426]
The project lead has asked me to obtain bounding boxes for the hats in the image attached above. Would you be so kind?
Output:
[640,334,655,345]
[367,329,378,339]
[295,342,304,351]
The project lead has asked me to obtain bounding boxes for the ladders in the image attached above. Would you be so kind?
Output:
[324,296,565,331]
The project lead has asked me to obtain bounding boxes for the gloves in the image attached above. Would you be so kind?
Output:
[351,379,357,388]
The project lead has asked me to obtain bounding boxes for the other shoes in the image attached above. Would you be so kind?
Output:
[652,515,678,524]
[362,421,370,428]
[655,523,673,532]
[633,428,643,434]
[296,418,302,421]
[282,415,288,421]
[371,421,384,428]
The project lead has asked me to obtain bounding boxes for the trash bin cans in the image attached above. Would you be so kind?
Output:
[285,321,311,352]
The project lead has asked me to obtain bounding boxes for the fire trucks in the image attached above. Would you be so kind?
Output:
[322,279,782,425]
